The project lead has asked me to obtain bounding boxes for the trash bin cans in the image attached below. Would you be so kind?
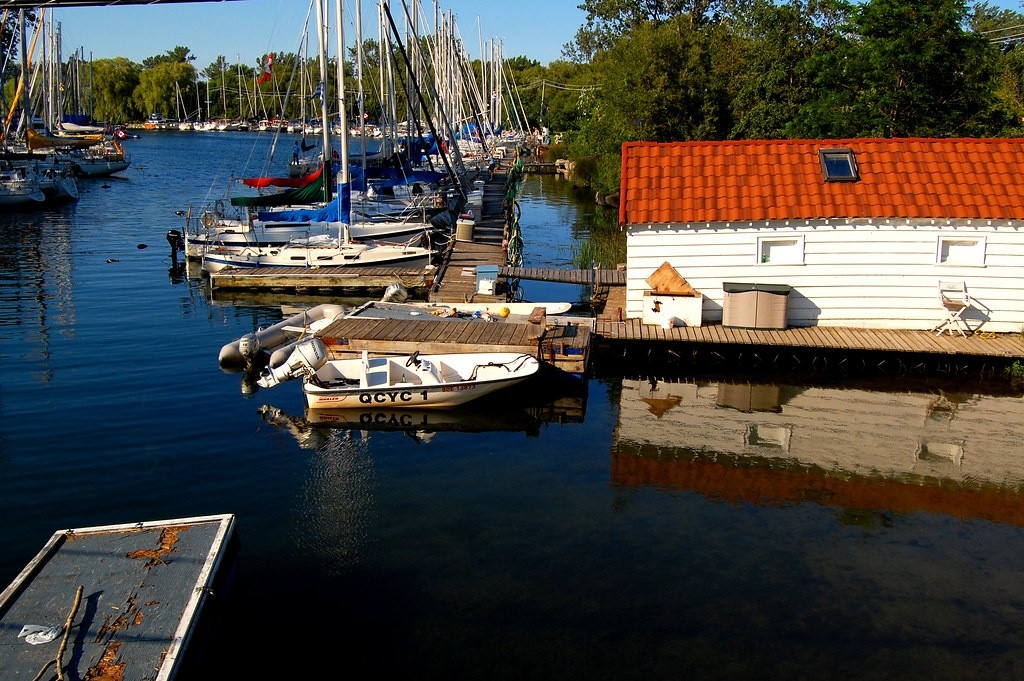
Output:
[717,385,782,414]
[720,283,790,331]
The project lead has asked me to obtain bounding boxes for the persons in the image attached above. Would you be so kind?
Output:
[505,125,560,146]
[485,152,495,181]
[292,140,301,165]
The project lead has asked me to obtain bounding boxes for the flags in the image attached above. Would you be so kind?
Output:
[257,52,276,85]
[307,81,324,105]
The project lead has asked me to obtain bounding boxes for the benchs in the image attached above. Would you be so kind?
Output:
[528,307,546,339]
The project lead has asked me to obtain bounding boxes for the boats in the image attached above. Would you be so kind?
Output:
[255,336,541,410]
[406,300,572,314]
[218,303,346,369]
[256,404,542,452]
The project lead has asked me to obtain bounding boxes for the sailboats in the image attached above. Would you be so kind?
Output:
[0,0,536,278]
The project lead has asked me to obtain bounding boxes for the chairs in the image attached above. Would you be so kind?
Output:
[930,280,970,339]
